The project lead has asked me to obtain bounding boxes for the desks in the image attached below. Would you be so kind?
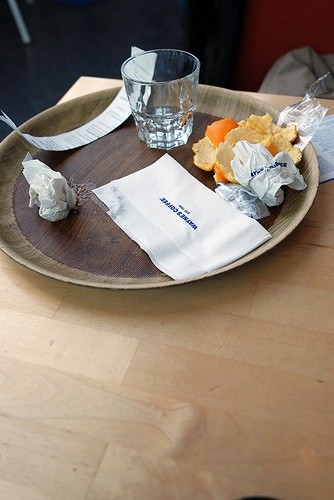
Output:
[0,73,334,500]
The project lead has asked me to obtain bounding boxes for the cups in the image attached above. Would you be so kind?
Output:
[120,49,201,150]
